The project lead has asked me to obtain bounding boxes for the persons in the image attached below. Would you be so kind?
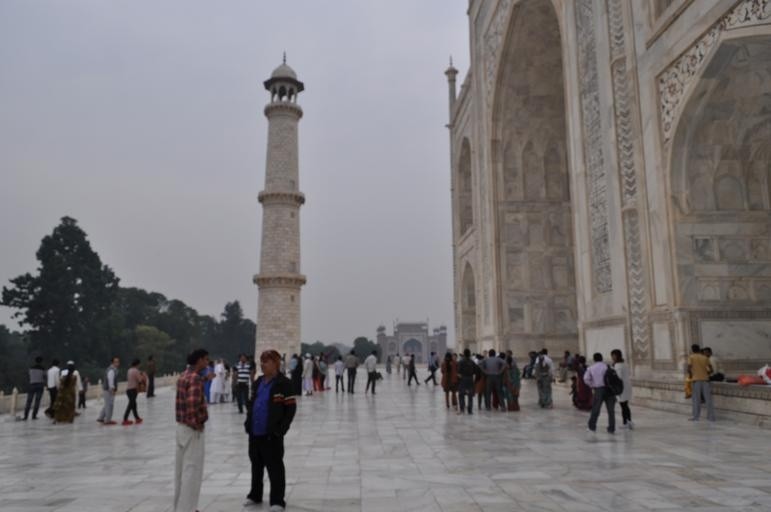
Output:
[240,349,298,512]
[200,349,257,414]
[171,347,216,512]
[19,353,157,426]
[700,346,725,403]
[687,343,716,422]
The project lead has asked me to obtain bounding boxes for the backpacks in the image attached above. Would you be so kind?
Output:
[604,365,623,396]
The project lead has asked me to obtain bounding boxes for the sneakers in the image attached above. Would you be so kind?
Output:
[122,420,133,425]
[242,498,256,506]
[105,420,117,425]
[135,419,143,424]
[270,505,284,512]
